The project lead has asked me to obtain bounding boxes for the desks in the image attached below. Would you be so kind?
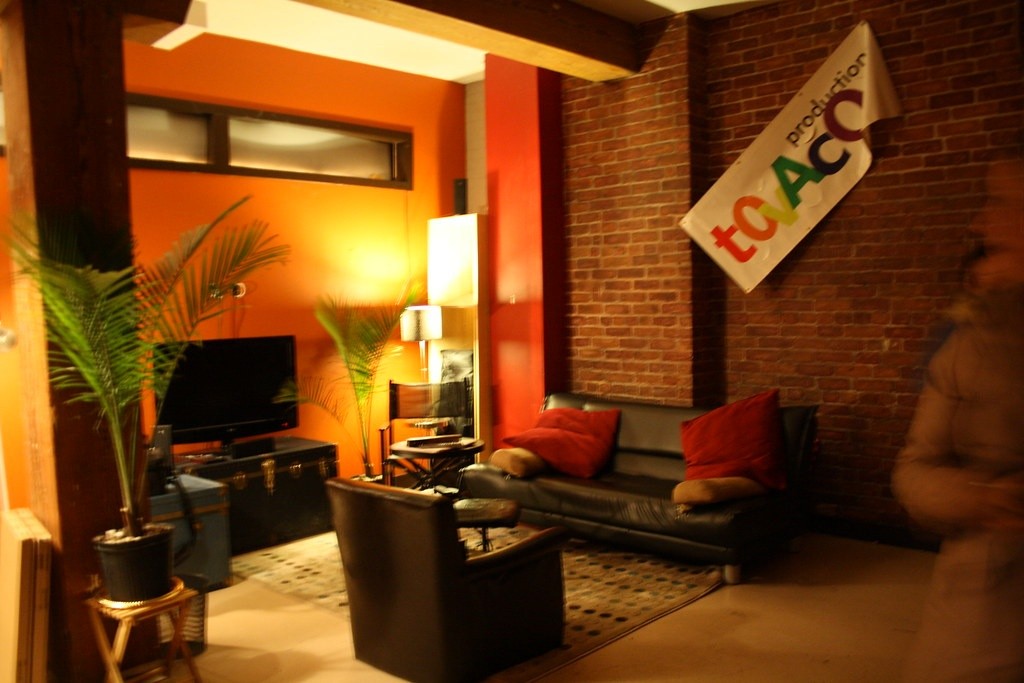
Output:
[84,587,200,683]
[390,435,486,490]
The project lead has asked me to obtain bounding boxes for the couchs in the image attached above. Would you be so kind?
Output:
[457,393,821,576]
[326,476,564,683]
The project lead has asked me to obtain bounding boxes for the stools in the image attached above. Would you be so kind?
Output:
[454,498,520,551]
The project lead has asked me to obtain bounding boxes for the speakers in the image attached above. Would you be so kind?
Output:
[229,438,276,459]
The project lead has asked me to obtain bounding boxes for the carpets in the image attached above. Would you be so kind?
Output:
[230,524,725,683]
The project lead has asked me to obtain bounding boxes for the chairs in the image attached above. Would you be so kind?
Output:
[378,380,473,487]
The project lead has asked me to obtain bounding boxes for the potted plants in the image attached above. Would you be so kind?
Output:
[0,192,293,604]
[272,278,430,484]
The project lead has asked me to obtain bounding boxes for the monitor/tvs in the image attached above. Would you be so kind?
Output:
[153,335,299,458]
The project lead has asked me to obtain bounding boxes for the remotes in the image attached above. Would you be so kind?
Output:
[434,485,454,496]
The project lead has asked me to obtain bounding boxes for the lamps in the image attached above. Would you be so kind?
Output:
[401,304,442,379]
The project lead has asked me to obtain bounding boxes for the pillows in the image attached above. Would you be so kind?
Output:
[679,386,787,490]
[673,477,771,513]
[501,407,622,478]
[491,447,551,476]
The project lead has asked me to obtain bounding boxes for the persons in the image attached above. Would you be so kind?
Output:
[894,134,1024,683]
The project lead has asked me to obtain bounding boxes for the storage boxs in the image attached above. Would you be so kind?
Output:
[170,436,339,559]
[147,473,232,591]
